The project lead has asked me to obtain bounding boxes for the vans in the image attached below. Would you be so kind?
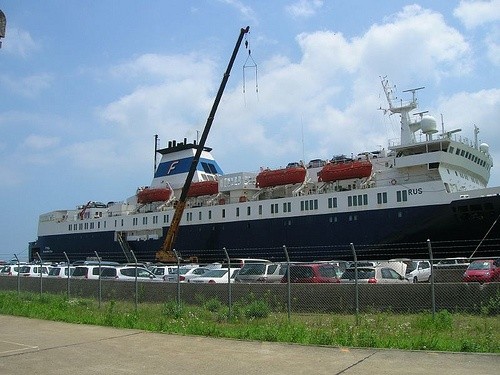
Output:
[221,258,273,268]
[70,266,115,279]
[0,265,22,276]
[47,266,75,278]
[97,266,164,281]
[18,265,53,277]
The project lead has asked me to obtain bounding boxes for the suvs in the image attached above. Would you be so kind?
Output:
[399,260,432,283]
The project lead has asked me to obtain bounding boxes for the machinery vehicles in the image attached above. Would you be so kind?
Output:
[149,26,259,265]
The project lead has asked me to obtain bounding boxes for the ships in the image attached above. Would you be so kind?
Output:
[28,75,500,265]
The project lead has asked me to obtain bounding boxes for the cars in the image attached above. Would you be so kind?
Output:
[234,263,288,283]
[280,264,340,283]
[125,263,148,270]
[151,266,181,278]
[188,268,241,283]
[433,257,471,269]
[163,266,206,283]
[336,266,412,283]
[463,259,500,285]
